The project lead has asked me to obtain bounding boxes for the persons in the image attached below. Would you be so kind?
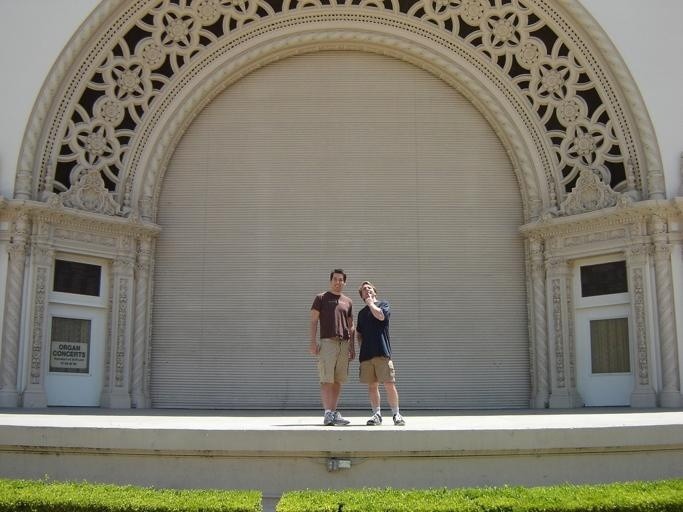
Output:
[308,268,355,425]
[354,280,406,426]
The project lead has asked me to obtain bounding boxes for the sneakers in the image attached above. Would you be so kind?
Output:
[392,413,405,426]
[332,411,350,425]
[323,412,335,426]
[366,413,382,426]
